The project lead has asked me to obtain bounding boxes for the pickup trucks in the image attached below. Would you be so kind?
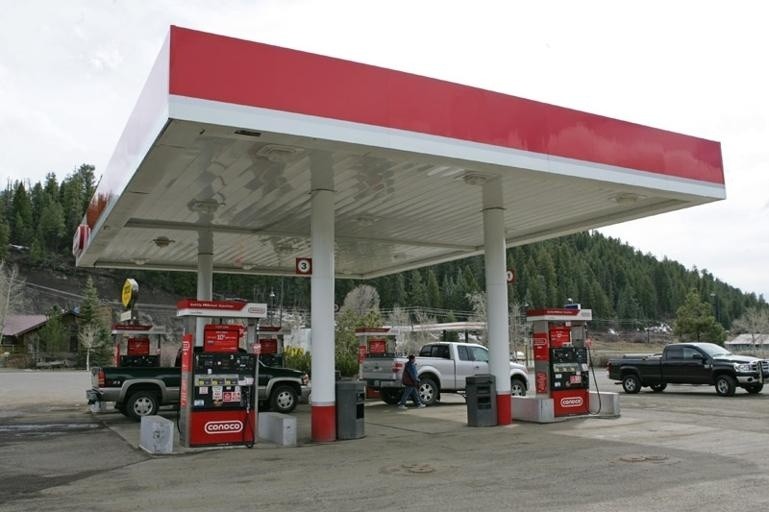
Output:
[359,341,530,406]
[606,342,769,397]
[86,347,310,422]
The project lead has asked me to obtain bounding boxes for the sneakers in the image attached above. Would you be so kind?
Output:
[398,404,408,409]
[417,402,426,408]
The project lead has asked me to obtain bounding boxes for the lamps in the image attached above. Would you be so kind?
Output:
[274,247,300,257]
[148,239,175,249]
[351,215,382,228]
[187,199,225,213]
[393,254,413,261]
[452,170,497,187]
[255,146,302,164]
[608,193,645,208]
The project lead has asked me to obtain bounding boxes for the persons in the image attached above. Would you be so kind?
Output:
[398,354,426,410]
